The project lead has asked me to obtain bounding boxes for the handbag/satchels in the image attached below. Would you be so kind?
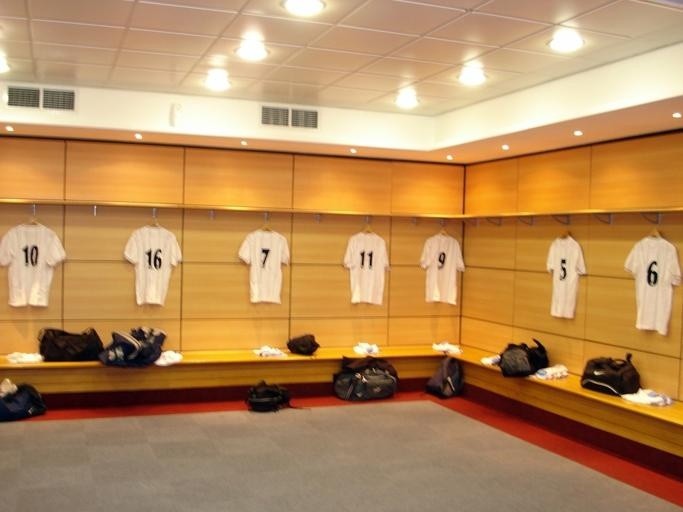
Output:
[0,383,46,422]
[99,326,167,369]
[497,337,549,378]
[580,353,640,397]
[38,327,104,362]
[288,334,319,355]
[335,356,400,401]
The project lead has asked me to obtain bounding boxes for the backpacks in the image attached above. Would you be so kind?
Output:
[246,381,291,411]
[426,357,462,398]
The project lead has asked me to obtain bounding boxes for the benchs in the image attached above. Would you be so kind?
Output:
[454,343,682,457]
[0,343,453,395]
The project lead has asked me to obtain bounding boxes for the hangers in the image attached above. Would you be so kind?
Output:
[130,208,174,238]
[426,219,458,243]
[633,218,675,250]
[244,213,286,241]
[3,204,58,237]
[549,220,579,248]
[348,216,384,242]
